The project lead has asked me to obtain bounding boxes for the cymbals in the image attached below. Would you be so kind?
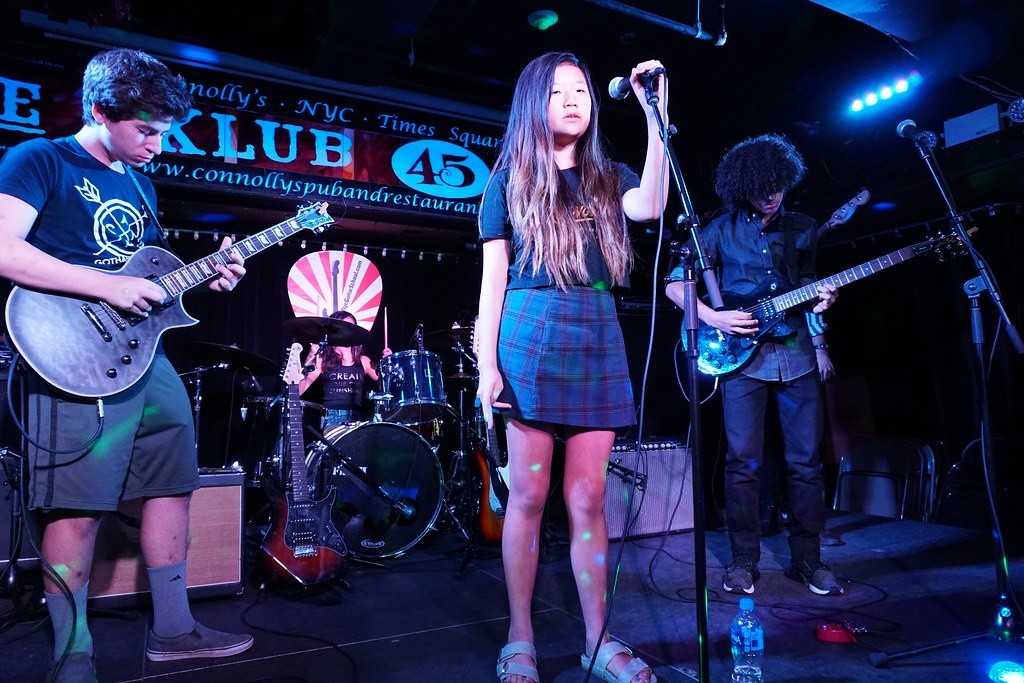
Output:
[418,325,476,340]
[281,313,373,350]
[191,339,279,371]
[440,369,477,383]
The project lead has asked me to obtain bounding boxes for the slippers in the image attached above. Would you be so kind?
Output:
[580,641,658,683]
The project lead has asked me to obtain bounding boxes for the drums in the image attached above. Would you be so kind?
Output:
[306,346,452,562]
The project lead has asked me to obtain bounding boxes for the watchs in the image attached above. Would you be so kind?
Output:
[813,343,829,350]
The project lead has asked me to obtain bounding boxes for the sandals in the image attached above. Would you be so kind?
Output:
[495,640,540,683]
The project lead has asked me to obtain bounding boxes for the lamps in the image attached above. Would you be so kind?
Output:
[916,97,1024,154]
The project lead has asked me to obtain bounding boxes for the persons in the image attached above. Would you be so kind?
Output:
[798,276,844,546]
[0,48,257,682]
[665,134,842,595]
[299,311,392,432]
[477,54,669,683]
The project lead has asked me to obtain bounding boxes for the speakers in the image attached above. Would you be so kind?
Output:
[86,467,246,611]
[0,445,42,573]
[605,435,693,541]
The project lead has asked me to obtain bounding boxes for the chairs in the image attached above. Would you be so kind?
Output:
[834,438,939,520]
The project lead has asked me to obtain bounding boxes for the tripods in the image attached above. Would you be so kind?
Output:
[1,449,45,625]
[868,142,1024,668]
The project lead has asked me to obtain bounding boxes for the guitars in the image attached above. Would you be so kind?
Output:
[254,342,348,585]
[465,316,532,547]
[680,201,1014,376]
[2,203,338,401]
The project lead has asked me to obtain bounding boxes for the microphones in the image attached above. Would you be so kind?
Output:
[248,369,263,393]
[608,68,666,99]
[638,476,648,491]
[410,323,423,349]
[897,119,930,146]
[400,504,416,519]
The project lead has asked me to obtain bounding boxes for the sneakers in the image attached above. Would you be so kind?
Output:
[785,559,845,595]
[722,554,761,594]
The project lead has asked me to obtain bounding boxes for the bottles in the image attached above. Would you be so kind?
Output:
[731,598,764,683]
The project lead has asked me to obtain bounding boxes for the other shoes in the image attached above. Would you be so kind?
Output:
[146,619,254,662]
[45,636,97,683]
[759,503,784,536]
[817,526,839,545]
